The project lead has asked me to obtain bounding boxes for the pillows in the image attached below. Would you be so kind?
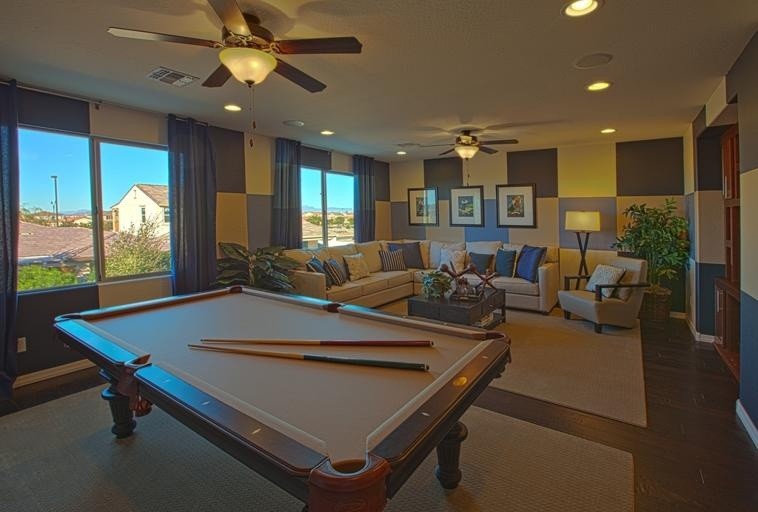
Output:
[517,245,547,283]
[305,254,333,291]
[387,241,426,270]
[469,251,494,274]
[493,246,518,279]
[341,252,370,281]
[323,255,347,286]
[615,282,631,300]
[586,264,626,298]
[379,248,407,271]
[438,247,467,273]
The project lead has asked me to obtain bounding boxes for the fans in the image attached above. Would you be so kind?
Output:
[419,130,520,155]
[107,1,362,93]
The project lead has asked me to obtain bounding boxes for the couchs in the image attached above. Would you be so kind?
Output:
[557,255,648,334]
[414,240,560,315]
[281,240,414,309]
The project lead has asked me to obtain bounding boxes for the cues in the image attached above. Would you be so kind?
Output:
[187,343,430,373]
[200,338,434,349]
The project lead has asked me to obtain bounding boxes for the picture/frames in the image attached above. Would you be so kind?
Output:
[496,183,536,228]
[407,187,439,226]
[448,185,484,227]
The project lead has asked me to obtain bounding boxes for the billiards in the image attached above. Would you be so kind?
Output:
[136,401,146,414]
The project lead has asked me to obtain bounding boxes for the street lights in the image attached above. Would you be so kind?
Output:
[49,173,61,227]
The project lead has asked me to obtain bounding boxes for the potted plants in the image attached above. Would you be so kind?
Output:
[422,271,454,300]
[609,197,691,323]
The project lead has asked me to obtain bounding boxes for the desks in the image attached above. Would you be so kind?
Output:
[53,283,511,511]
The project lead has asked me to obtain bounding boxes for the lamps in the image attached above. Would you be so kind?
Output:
[564,211,600,290]
[220,47,277,147]
[455,145,479,187]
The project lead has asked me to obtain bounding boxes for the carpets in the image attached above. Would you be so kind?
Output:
[374,298,648,428]
[1,382,634,512]
